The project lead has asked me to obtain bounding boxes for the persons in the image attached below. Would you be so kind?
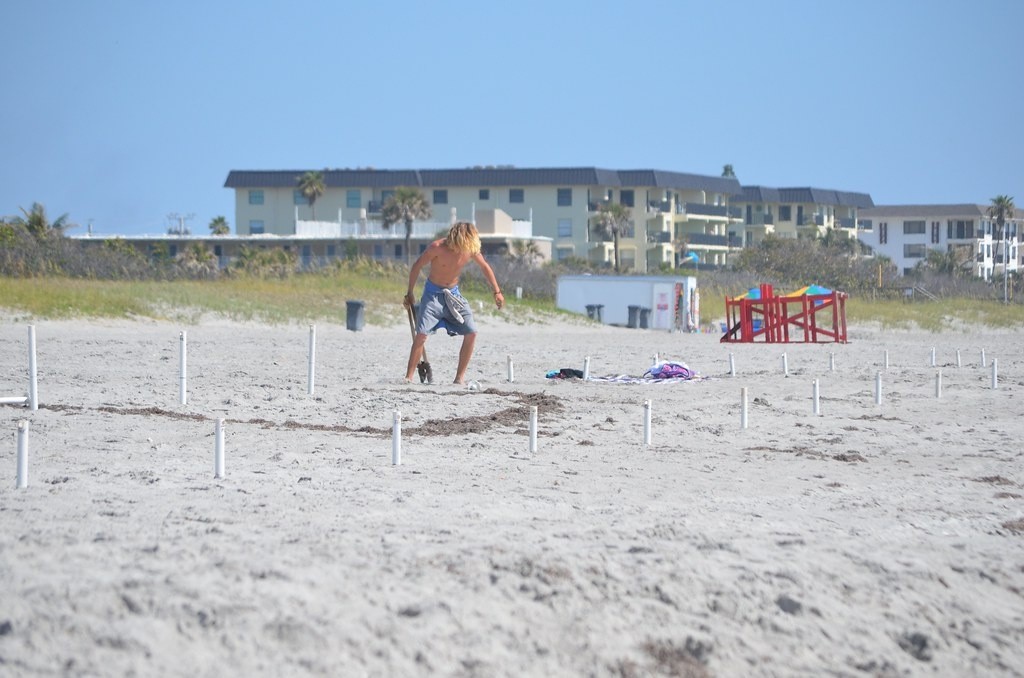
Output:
[403,221,504,385]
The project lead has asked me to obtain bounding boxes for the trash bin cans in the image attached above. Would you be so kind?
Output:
[346,300,366,333]
[585,304,605,324]
[627,304,641,328]
[640,308,652,328]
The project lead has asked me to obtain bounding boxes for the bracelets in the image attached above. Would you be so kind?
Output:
[494,291,501,295]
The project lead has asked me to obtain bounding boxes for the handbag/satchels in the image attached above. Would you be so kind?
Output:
[643,363,696,380]
